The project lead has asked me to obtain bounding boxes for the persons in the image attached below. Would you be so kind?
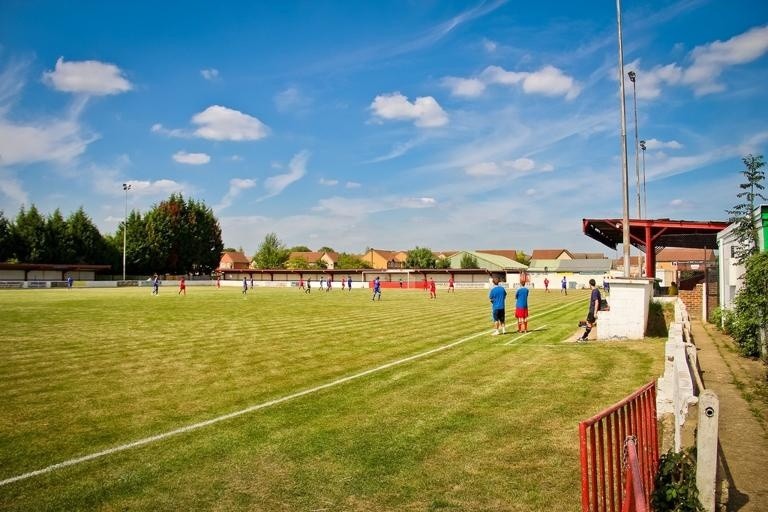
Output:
[150,276,161,296]
[178,276,186,295]
[667,281,677,295]
[428,276,436,299]
[560,276,567,296]
[654,285,661,296]
[305,277,311,295]
[514,279,530,333]
[340,276,346,290]
[242,276,248,294]
[65,274,73,290]
[216,273,220,288]
[348,276,352,292]
[611,276,613,279]
[603,276,606,291]
[250,275,254,289]
[326,276,331,292]
[371,276,381,300]
[578,279,601,341]
[298,276,305,291]
[399,278,403,288]
[319,277,324,290]
[605,276,610,293]
[447,276,455,293]
[488,277,508,336]
[423,279,428,292]
[543,278,550,292]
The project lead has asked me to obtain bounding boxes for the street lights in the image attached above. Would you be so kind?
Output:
[121,183,132,279]
[625,70,643,278]
[638,139,648,218]
